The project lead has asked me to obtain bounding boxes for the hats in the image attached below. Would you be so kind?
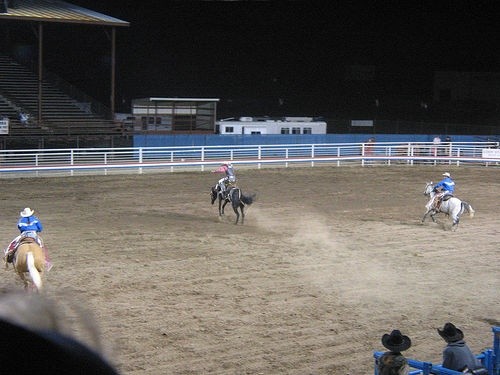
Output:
[21,207,34,217]
[382,330,411,351]
[437,322,464,342]
[442,172,450,178]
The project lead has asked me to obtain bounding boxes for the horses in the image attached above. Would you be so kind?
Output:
[209,182,253,225]
[420,180,475,232]
[6,230,46,293]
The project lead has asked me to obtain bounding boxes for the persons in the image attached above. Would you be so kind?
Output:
[215,161,236,199]
[377,329,412,375]
[432,135,452,153]
[3,207,52,270]
[432,172,455,213]
[363,137,375,163]
[438,322,476,375]
